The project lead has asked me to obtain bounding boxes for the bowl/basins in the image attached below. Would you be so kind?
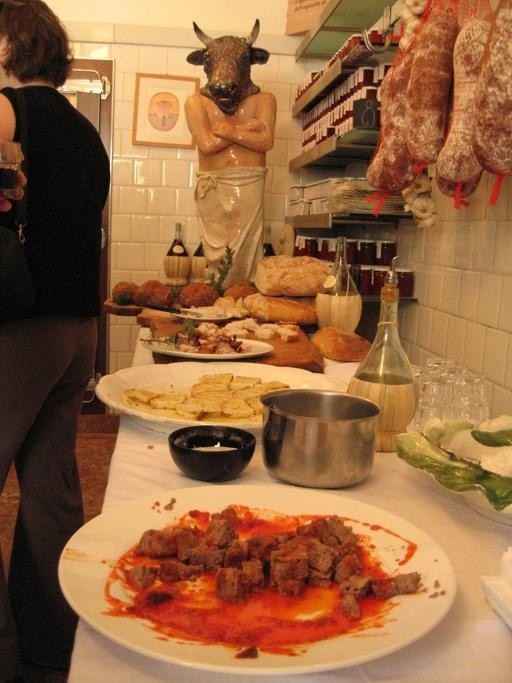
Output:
[168,425,255,482]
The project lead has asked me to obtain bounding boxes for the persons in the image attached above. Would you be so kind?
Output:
[0,152,27,212]
[184,91,277,291]
[0,0,110,683]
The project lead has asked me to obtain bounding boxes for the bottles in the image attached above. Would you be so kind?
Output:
[351,255,418,453]
[264,224,276,256]
[165,222,192,286]
[319,234,362,334]
[190,238,211,283]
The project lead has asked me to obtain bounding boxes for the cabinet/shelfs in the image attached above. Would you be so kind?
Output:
[284,0,419,305]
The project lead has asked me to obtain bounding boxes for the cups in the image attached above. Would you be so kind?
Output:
[415,350,490,434]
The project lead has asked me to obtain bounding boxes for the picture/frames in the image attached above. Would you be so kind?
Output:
[131,72,201,150]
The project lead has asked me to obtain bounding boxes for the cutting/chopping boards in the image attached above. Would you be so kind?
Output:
[149,315,326,372]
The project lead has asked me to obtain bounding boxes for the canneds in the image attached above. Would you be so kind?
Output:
[296,237,414,298]
[295,33,393,153]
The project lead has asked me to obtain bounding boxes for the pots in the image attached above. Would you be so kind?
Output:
[259,390,381,490]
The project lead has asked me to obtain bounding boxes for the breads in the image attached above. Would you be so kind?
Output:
[109,255,373,374]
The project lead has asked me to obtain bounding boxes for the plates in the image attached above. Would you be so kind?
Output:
[59,482,459,674]
[174,313,234,323]
[143,338,272,358]
[420,465,512,525]
[98,362,349,429]
